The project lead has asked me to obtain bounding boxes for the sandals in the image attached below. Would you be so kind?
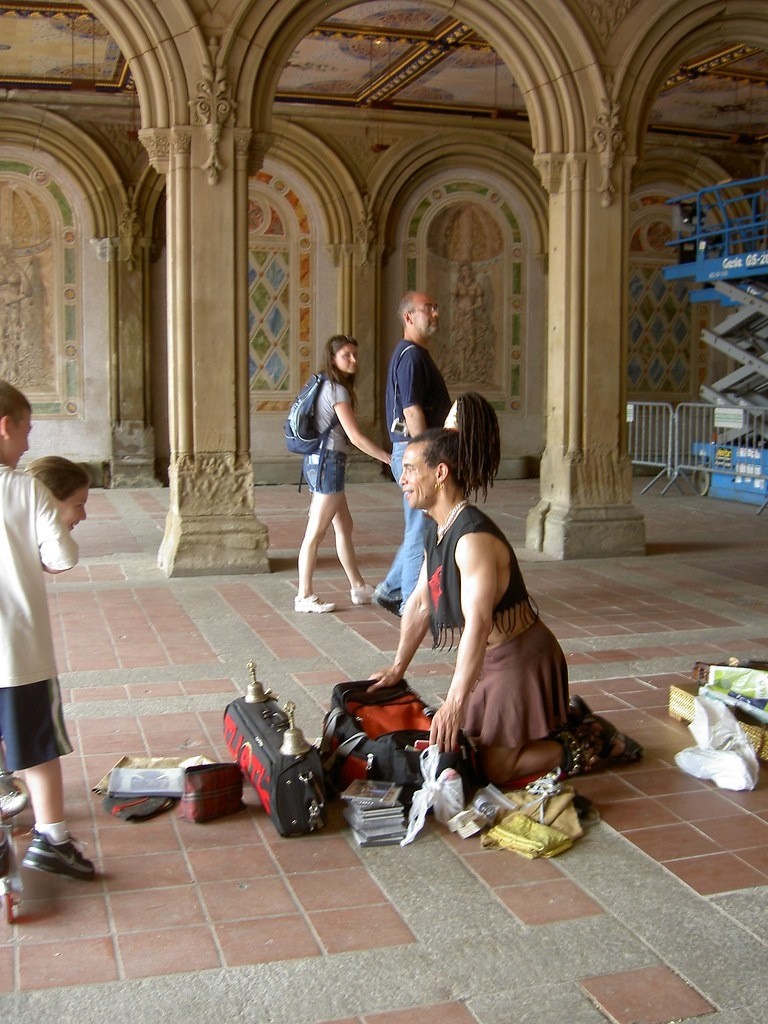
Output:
[566,694,590,728]
[584,715,645,765]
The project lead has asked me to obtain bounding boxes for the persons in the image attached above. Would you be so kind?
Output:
[372,291,452,619]
[367,392,644,786]
[295,335,392,613]
[0,379,95,882]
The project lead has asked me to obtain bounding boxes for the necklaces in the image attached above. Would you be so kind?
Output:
[437,499,469,536]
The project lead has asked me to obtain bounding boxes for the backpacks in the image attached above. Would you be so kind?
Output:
[283,371,340,454]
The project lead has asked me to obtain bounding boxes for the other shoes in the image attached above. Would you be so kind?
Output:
[351,583,375,604]
[294,593,336,612]
[377,596,402,616]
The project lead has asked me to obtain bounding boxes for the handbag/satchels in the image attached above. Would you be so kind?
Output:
[675,696,760,790]
[691,661,768,723]
[318,677,479,813]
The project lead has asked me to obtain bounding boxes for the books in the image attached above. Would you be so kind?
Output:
[341,778,408,848]
[107,768,185,796]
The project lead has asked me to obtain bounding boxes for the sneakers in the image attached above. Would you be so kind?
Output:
[23,831,95,882]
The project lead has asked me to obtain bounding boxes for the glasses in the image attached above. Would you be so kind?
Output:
[334,334,351,343]
[409,305,439,315]
